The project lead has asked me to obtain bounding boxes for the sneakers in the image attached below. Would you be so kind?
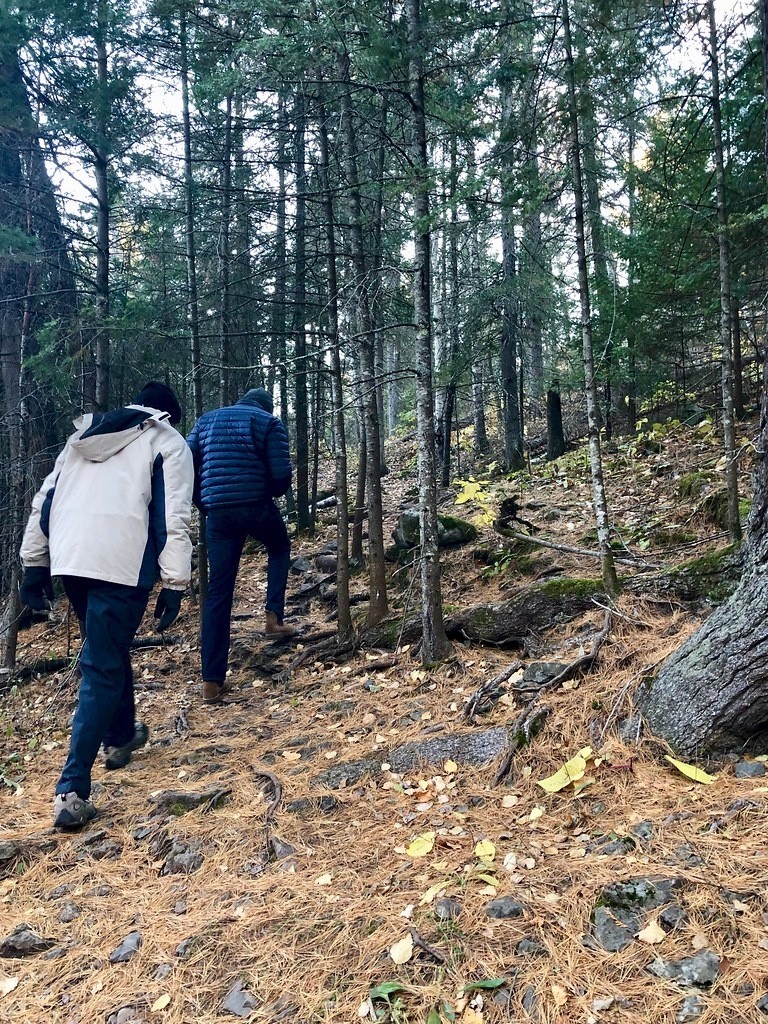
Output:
[52,792,96,829]
[103,722,149,770]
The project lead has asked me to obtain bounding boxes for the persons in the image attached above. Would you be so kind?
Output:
[20,381,193,828]
[185,387,294,704]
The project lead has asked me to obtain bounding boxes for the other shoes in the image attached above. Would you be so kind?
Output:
[202,681,232,704]
[264,610,297,640]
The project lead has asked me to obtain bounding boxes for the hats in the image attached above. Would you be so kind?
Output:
[242,386,274,412]
[132,381,181,425]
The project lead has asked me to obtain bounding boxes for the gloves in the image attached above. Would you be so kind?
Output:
[19,566,54,610]
[154,588,183,633]
